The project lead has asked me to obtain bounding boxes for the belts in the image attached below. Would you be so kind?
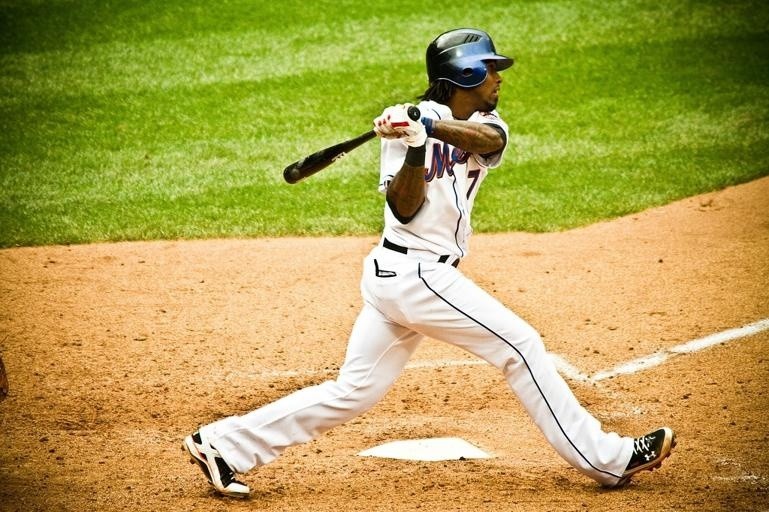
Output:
[379,237,461,270]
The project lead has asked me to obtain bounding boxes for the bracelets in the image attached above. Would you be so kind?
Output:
[404,144,425,167]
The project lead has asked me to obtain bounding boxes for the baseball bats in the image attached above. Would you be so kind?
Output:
[283,107,420,184]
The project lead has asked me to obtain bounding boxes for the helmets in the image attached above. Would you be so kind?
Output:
[426,29,514,89]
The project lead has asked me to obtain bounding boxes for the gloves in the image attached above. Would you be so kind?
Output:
[373,101,402,139]
[386,103,428,148]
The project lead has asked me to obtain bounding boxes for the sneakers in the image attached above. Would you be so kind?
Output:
[180,427,250,499]
[615,428,676,488]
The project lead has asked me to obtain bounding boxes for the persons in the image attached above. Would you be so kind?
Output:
[178,28,676,499]
